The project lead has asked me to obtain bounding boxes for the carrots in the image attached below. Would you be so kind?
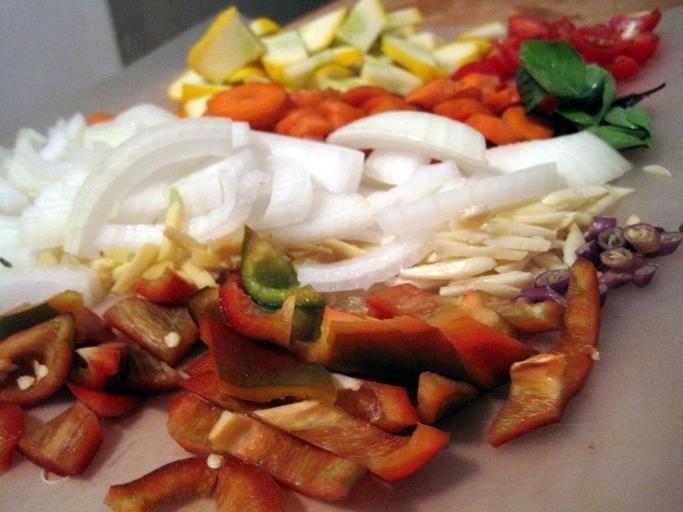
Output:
[203,74,553,143]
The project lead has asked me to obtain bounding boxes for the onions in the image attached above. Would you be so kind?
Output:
[0,103,635,315]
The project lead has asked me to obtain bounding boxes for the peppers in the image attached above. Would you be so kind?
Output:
[0,225,600,512]
